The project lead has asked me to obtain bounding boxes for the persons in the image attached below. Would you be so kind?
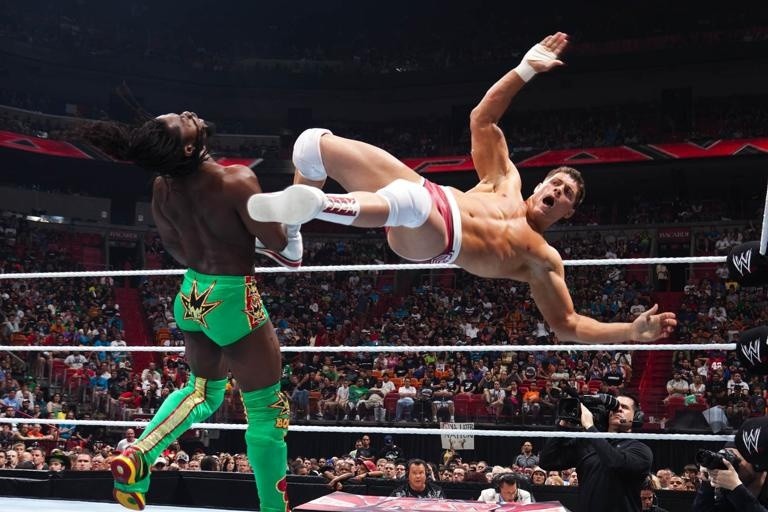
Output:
[113,109,292,512]
[1,212,767,512]
[245,32,675,342]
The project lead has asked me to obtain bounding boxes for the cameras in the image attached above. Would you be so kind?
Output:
[694,448,740,474]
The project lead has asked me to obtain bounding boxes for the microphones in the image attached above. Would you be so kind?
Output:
[620,418,639,424]
[425,479,430,485]
[514,488,518,502]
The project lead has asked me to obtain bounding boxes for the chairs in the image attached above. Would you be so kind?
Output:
[385,393,400,419]
[520,380,546,395]
[590,380,602,391]
[53,360,88,395]
[391,378,403,387]
[454,392,488,420]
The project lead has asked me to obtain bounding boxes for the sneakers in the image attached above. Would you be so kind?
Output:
[108,442,150,512]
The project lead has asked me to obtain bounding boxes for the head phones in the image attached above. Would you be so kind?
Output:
[627,393,645,429]
[405,459,432,479]
[494,472,520,493]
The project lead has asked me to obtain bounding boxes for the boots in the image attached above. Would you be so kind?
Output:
[254,220,305,270]
[243,181,361,229]
